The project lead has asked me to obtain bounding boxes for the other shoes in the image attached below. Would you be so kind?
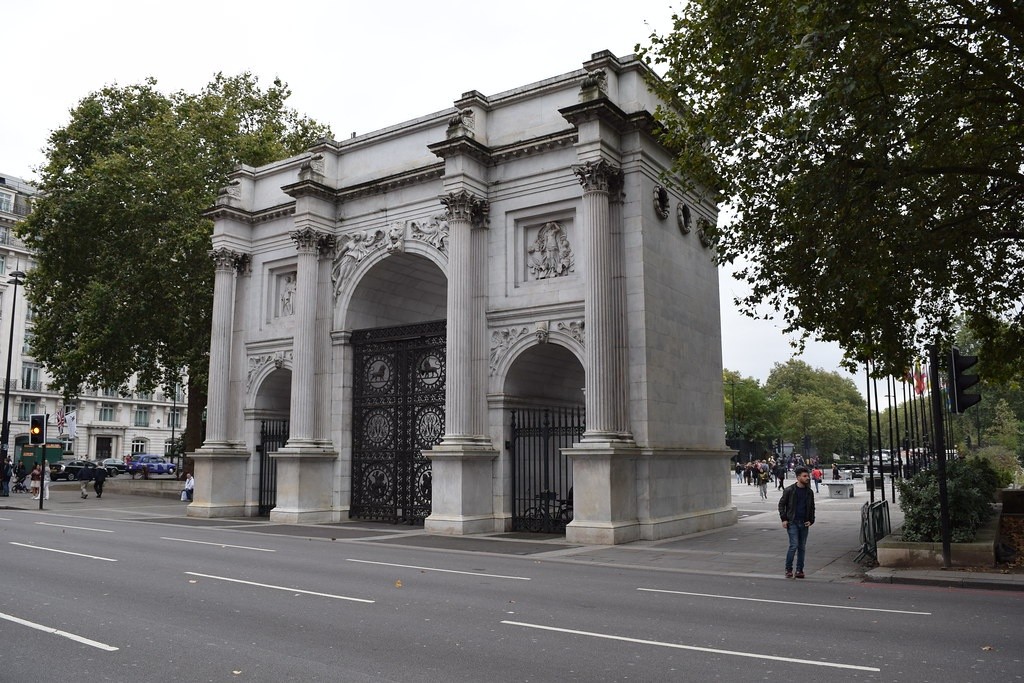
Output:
[186,500,191,502]
[0,494,9,496]
[81,497,84,498]
[32,494,40,500]
[84,495,88,499]
[794,570,804,578]
[785,568,793,577]
[96,495,101,498]
[44,496,49,500]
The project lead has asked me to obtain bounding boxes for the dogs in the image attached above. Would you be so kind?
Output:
[12,484,28,494]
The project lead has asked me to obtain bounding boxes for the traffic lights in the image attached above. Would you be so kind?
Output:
[29,414,46,445]
[949,343,982,415]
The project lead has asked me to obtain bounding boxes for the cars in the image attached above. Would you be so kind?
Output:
[127,454,177,474]
[50,459,98,481]
[91,458,128,477]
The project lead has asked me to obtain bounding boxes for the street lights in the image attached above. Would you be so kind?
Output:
[722,381,744,434]
[0,258,28,489]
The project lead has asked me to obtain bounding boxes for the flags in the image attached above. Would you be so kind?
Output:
[65,412,77,439]
[900,361,932,394]
[56,409,65,434]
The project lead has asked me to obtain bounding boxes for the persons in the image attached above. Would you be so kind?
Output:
[126,454,131,464]
[0,455,51,500]
[181,473,194,501]
[77,463,91,499]
[416,217,450,252]
[542,222,561,274]
[281,276,294,316]
[812,467,822,493]
[778,468,815,579]
[757,468,768,500]
[832,463,839,480]
[735,455,804,491]
[93,461,107,498]
[333,233,376,297]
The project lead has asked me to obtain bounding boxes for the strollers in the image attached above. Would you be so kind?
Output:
[12,473,30,493]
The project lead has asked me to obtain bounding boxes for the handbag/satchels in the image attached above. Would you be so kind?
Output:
[819,479,822,483]
[180,491,188,501]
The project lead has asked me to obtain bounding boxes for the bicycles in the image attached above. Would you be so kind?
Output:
[523,495,573,533]
